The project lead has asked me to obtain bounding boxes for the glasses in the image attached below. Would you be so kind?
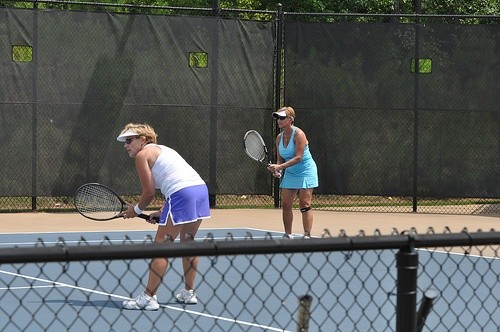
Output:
[277,117,287,120]
[125,136,140,144]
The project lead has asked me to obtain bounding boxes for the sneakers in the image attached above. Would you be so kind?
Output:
[122,292,159,311]
[176,289,197,304]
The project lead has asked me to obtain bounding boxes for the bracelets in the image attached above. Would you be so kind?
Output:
[134,203,143,215]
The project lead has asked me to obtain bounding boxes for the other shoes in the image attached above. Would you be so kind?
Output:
[282,234,294,240]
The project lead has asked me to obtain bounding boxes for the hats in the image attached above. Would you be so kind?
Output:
[272,110,294,122]
[117,129,139,142]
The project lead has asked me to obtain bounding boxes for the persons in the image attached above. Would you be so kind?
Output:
[117,124,211,310]
[267,107,318,240]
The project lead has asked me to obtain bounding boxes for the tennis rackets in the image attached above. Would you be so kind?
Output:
[417,289,436,332]
[74,183,161,224]
[242,129,281,176]
[297,294,313,332]
